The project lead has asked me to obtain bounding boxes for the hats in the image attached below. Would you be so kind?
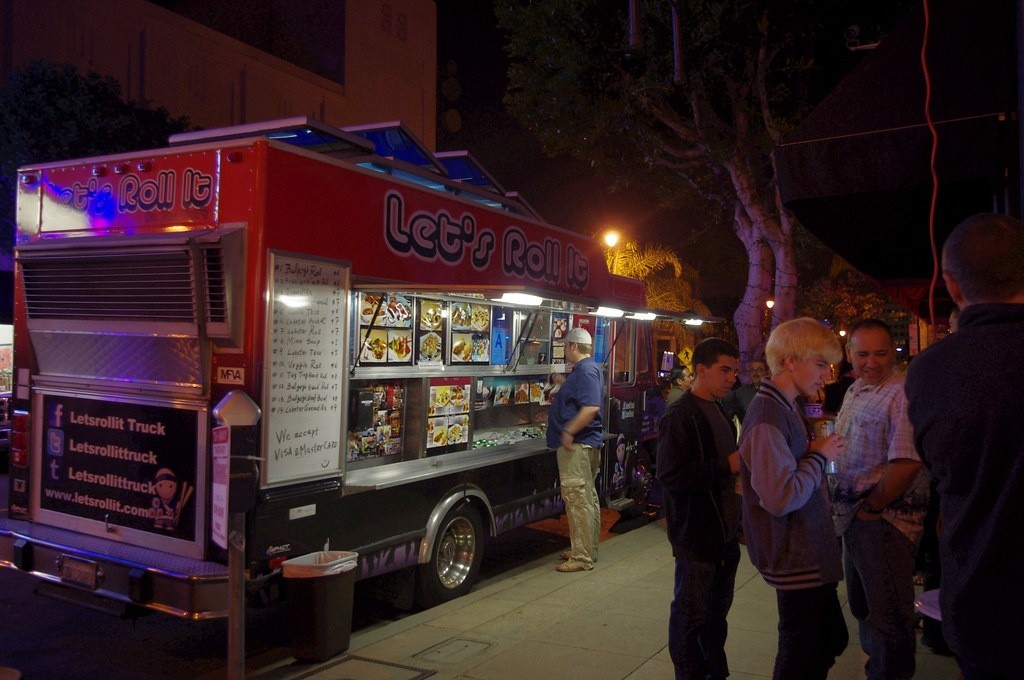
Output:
[557,327,592,345]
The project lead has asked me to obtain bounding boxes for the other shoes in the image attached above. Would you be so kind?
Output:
[557,560,584,572]
[560,551,596,562]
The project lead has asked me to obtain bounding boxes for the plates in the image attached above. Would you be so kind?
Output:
[473,381,555,409]
[426,383,469,448]
[359,290,492,374]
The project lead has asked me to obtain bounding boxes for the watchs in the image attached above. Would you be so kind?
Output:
[862,499,885,515]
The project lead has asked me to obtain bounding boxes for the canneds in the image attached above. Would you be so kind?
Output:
[813,420,838,475]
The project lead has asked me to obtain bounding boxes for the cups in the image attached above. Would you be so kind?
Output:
[619,371,628,383]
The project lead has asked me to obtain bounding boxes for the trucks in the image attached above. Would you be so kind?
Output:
[0,116,726,622]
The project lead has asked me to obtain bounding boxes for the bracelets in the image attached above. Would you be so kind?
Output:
[562,429,574,437]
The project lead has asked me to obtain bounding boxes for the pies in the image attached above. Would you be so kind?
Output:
[393,337,406,359]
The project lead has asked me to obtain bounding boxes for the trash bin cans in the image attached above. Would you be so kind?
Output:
[913,589,954,657]
[280,551,358,662]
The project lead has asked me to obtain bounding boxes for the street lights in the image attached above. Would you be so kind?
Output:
[606,235,621,274]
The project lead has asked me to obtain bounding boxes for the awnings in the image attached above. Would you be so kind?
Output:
[598,300,726,376]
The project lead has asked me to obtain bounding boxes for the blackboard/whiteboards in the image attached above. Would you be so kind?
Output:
[259,246,350,491]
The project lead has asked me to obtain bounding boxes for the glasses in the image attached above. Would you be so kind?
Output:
[746,367,767,374]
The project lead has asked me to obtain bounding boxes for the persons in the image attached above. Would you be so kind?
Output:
[825,318,927,680]
[648,380,673,430]
[666,366,695,408]
[656,337,745,680]
[546,327,605,572]
[729,358,772,429]
[904,211,1024,680]
[738,315,849,680]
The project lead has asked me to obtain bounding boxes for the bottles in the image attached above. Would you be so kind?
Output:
[657,350,684,386]
[472,423,546,449]
